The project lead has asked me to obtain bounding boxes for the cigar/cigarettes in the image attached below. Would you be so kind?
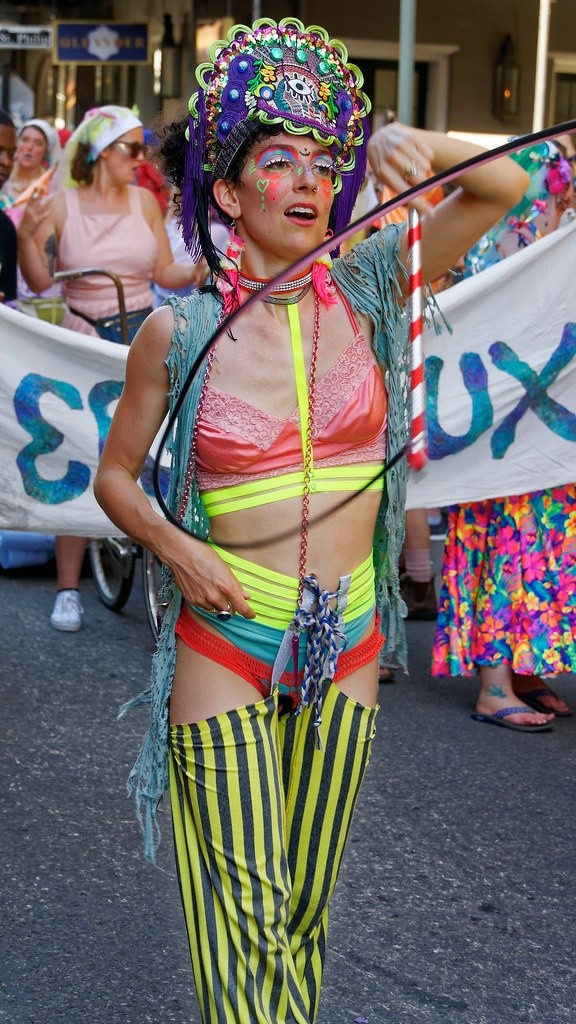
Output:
[33,187,43,201]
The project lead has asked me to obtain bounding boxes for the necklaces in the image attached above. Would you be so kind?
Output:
[235,268,319,307]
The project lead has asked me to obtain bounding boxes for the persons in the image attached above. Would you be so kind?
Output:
[1,111,65,309]
[430,130,575,732]
[395,504,440,623]
[88,17,530,1023]
[20,103,213,630]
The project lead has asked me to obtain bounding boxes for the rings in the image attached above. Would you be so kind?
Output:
[402,161,417,182]
[216,602,232,620]
[203,607,217,614]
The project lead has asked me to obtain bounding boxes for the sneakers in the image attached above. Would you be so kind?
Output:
[399,576,438,620]
[49,589,82,632]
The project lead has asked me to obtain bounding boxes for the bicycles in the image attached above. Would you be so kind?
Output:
[50,266,183,651]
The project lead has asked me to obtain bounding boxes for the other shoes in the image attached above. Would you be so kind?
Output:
[378,673,395,679]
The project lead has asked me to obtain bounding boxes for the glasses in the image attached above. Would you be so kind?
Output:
[113,140,147,158]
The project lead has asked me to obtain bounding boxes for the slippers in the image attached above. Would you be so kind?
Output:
[471,706,555,732]
[514,688,572,715]
